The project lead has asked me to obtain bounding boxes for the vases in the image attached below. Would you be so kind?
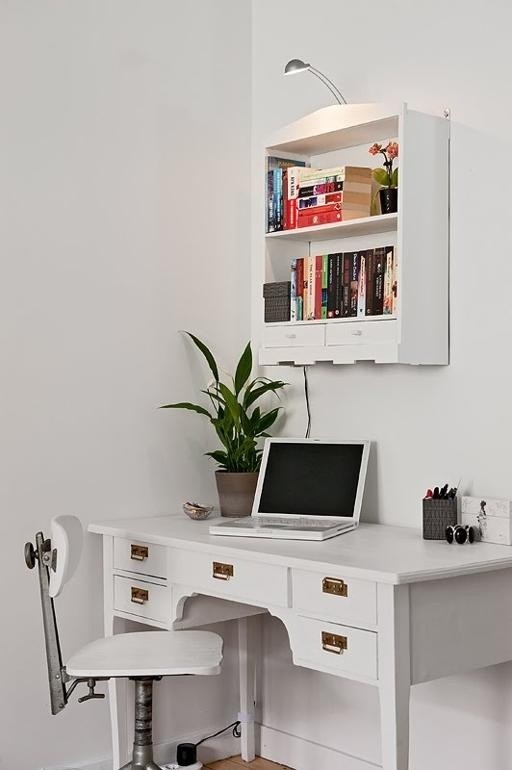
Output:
[215,473,260,516]
[380,188,396,213]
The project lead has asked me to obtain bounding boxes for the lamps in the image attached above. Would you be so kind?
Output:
[285,59,347,105]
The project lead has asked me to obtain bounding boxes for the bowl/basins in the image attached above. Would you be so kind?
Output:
[183,503,214,521]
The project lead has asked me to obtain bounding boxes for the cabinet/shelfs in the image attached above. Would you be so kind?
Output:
[258,102,449,367]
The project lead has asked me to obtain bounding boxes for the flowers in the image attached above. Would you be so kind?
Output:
[370,142,399,215]
[155,330,288,473]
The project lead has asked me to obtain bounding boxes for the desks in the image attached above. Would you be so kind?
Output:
[87,505,512,769]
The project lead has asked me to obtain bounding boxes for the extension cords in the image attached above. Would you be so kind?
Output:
[160,760,203,770]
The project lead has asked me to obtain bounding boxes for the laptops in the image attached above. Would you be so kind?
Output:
[208,437,371,540]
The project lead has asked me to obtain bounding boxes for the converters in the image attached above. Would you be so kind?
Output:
[177,743,197,766]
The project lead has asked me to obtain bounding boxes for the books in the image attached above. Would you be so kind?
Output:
[288,244,399,321]
[265,154,377,233]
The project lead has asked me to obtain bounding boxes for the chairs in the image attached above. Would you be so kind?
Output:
[26,515,225,768]
